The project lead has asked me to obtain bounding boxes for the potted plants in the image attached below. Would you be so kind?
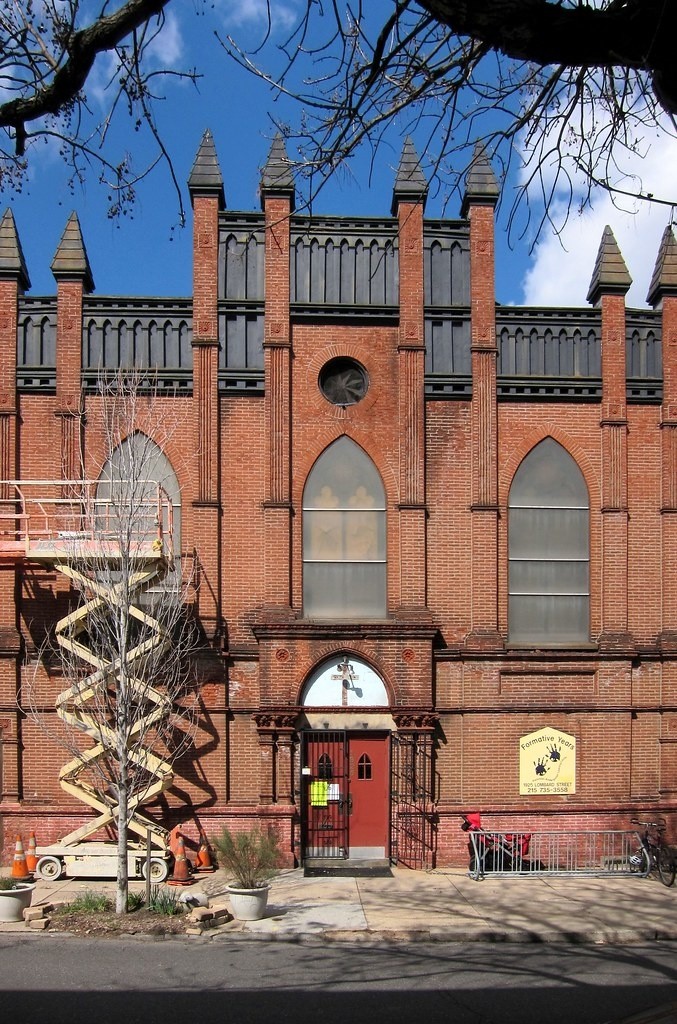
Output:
[207,822,285,919]
[0,875,36,923]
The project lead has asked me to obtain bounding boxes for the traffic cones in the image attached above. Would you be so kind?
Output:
[163,835,197,886]
[25,830,40,873]
[193,828,213,873]
[8,836,32,881]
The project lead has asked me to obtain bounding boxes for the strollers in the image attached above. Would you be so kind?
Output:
[461,813,538,873]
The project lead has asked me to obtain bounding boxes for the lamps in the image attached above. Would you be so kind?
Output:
[337,662,355,675]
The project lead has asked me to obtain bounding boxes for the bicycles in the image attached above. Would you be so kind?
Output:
[628,817,676,886]
